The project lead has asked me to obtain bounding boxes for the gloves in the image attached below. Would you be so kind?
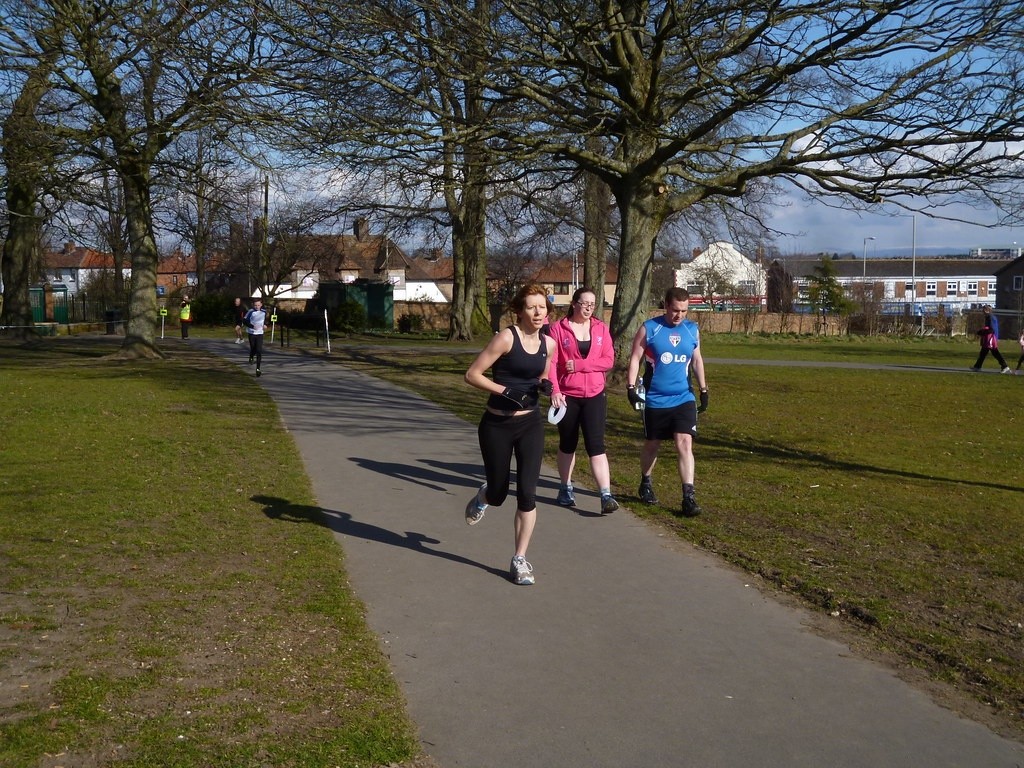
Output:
[501,386,529,410]
[697,392,708,414]
[628,389,646,412]
[542,379,554,396]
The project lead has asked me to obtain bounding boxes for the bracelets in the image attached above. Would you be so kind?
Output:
[626,385,636,388]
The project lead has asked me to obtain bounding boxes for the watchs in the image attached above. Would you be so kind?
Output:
[699,387,709,391]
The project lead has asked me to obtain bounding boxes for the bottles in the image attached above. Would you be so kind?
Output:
[635,376,645,410]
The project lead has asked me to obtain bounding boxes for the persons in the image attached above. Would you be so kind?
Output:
[464,285,556,584]
[549,288,619,515]
[970,305,1010,374]
[179,294,192,339]
[243,299,267,377]
[627,287,709,517]
[233,298,244,344]
[1014,329,1024,374]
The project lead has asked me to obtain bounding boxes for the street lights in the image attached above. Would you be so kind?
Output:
[890,213,916,315]
[863,237,875,287]
[759,228,771,312]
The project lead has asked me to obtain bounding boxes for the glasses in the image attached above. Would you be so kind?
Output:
[576,302,596,309]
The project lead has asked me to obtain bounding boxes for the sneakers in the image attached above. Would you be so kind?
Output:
[683,492,702,516]
[510,556,535,585]
[638,484,658,504]
[601,496,620,514]
[557,489,575,505]
[465,483,488,526]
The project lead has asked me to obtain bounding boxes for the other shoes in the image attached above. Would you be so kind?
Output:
[235,338,239,343]
[999,366,1010,374]
[256,369,261,377]
[184,337,188,339]
[970,366,984,373]
[238,340,244,344]
[1014,370,1019,374]
[249,358,254,365]
